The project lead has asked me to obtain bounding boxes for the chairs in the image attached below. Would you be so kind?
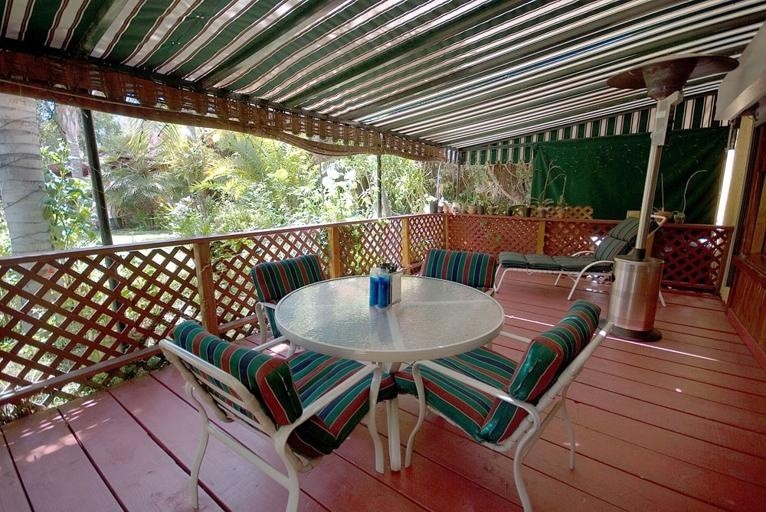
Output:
[250,253,326,345]
[394,298,614,512]
[159,320,396,512]
[494,215,667,307]
[419,249,496,297]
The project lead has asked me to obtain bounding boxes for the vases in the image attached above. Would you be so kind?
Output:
[429,200,485,215]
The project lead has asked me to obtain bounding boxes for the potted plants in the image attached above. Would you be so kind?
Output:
[672,170,707,225]
[652,172,673,221]
[487,199,496,216]
